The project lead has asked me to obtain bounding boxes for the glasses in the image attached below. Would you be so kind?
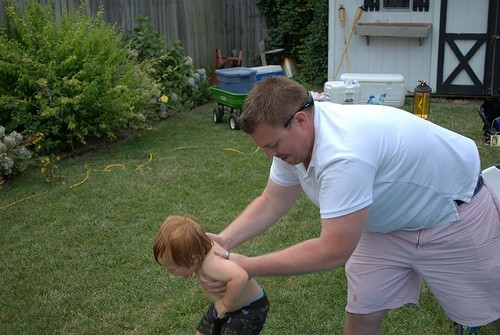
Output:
[284,91,314,128]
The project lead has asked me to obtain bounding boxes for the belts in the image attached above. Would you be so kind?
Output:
[455,176,484,206]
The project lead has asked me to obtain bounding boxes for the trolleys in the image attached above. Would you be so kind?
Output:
[208,86,249,130]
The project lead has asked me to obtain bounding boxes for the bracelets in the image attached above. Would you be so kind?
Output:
[221,299,231,311]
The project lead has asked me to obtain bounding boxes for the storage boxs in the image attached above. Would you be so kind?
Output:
[215,65,283,93]
[340,74,405,107]
[324,80,361,104]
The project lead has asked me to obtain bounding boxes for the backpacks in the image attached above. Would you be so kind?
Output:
[478,98,500,144]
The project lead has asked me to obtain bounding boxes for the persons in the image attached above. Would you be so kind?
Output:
[153,214,270,335]
[206,75,500,335]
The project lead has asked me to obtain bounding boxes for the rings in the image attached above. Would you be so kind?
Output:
[225,251,231,258]
